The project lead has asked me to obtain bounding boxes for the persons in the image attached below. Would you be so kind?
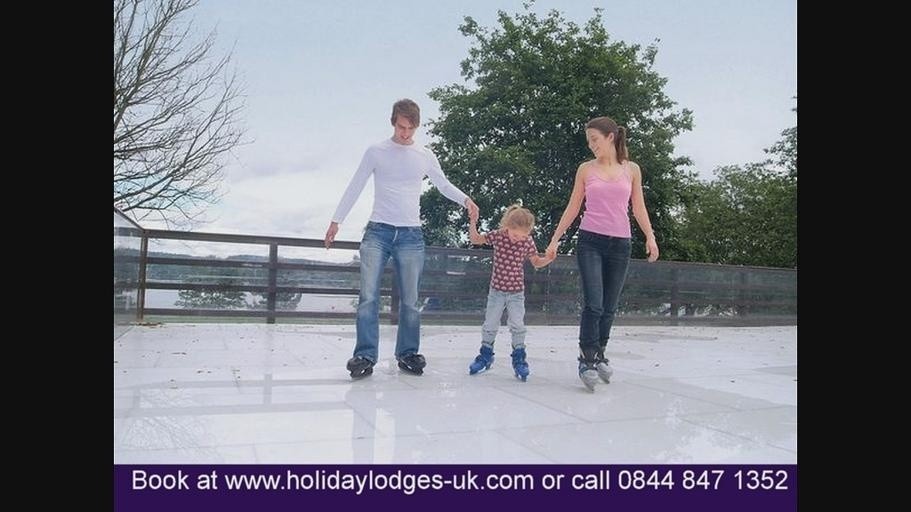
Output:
[546,118,659,384]
[324,98,478,371]
[469,204,557,377]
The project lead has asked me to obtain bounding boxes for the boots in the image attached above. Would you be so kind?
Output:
[511,348,529,376]
[577,346,598,381]
[469,344,494,373]
[595,346,613,378]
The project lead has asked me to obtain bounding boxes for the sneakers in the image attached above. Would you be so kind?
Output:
[347,356,374,370]
[396,352,426,369]
